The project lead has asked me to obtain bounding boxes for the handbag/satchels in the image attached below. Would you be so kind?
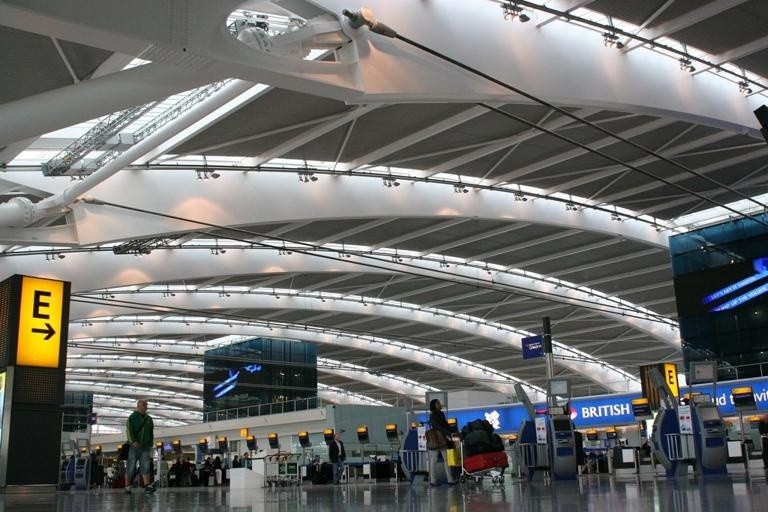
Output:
[465,430,505,455]
[425,428,448,450]
[446,448,462,466]
[118,443,131,460]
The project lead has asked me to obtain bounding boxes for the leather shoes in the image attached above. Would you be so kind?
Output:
[333,481,340,485]
[429,481,441,487]
[448,480,459,486]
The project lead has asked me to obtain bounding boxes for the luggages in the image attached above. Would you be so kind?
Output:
[463,452,509,472]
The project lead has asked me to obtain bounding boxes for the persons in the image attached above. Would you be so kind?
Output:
[328,432,346,485]
[88,448,252,489]
[426,398,460,488]
[757,414,767,469]
[121,398,157,496]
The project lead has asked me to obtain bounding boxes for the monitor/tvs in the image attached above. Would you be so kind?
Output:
[553,419,571,431]
[76,459,85,465]
[63,461,69,466]
[649,368,666,388]
[698,406,721,421]
[78,439,89,447]
[547,378,572,396]
[514,384,525,401]
[689,360,718,383]
[653,410,663,425]
[426,392,447,407]
[63,442,71,450]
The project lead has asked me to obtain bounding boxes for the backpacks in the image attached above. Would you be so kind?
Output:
[459,419,494,440]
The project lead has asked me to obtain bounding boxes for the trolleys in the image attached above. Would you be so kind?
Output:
[448,432,510,486]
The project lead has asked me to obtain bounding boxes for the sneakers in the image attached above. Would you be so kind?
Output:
[145,483,156,493]
[125,485,133,496]
